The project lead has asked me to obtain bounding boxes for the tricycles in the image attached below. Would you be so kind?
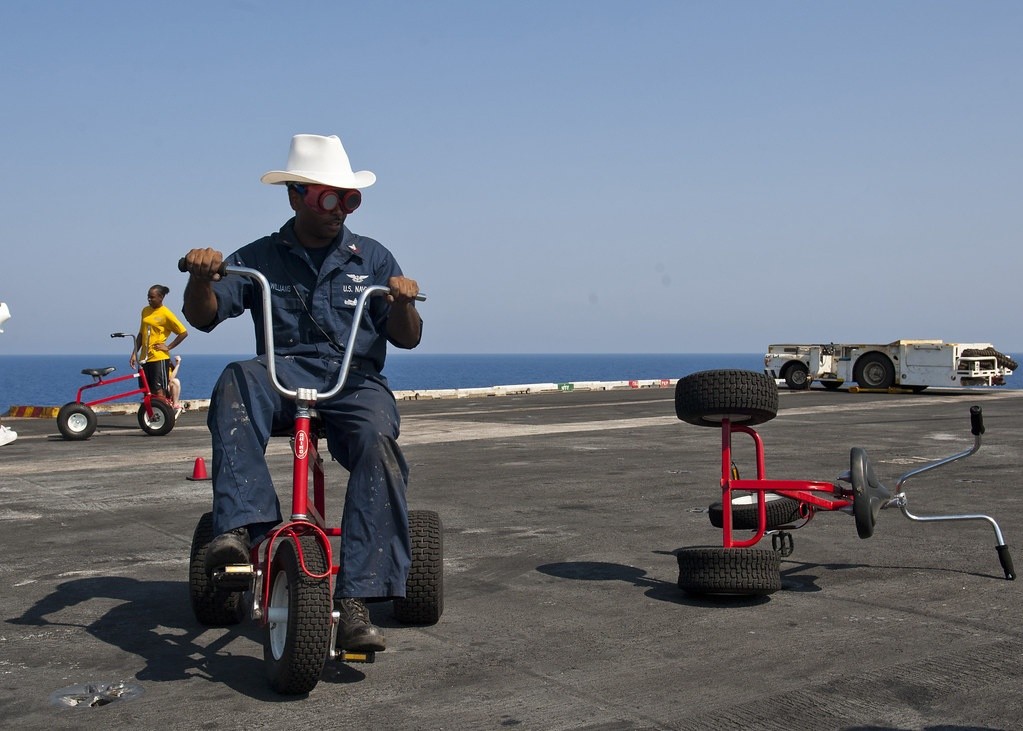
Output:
[56,333,176,440]
[177,257,444,695]
[675,368,1016,598]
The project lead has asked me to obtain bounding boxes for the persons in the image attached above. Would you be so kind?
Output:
[129,285,188,397]
[168,356,185,420]
[0,302,16,447]
[183,134,422,651]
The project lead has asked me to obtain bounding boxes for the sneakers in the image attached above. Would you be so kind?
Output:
[331,599,387,653]
[206,529,253,591]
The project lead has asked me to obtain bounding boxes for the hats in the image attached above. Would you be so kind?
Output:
[260,133,377,188]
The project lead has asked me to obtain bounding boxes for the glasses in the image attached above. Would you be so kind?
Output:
[285,180,363,215]
